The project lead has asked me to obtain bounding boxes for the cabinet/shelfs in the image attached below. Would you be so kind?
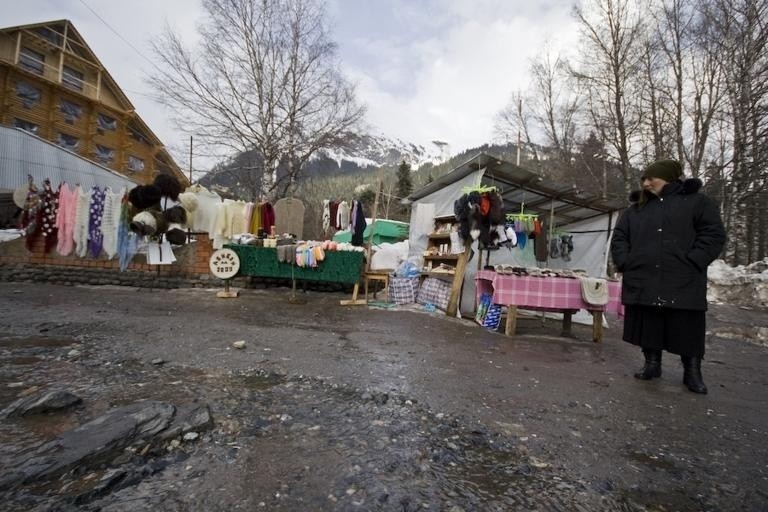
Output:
[419,214,473,317]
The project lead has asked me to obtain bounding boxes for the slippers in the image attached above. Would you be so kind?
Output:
[494,264,578,278]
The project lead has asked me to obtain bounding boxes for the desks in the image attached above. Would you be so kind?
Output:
[223,242,366,306]
[364,271,390,305]
[472,269,622,344]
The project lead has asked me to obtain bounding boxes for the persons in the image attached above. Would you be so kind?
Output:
[609,158,729,397]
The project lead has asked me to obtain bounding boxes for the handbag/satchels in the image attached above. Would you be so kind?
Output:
[387,273,419,306]
[474,294,502,329]
[417,278,452,312]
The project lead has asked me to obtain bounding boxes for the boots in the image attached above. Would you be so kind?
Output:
[681,354,707,393]
[634,347,662,379]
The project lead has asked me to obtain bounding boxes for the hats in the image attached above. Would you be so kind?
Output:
[128,174,198,245]
[641,159,682,183]
